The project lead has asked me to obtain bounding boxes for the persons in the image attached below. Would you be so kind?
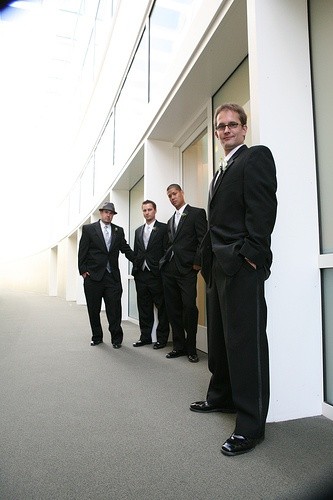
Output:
[159,184,208,363]
[189,103,278,455]
[132,199,169,349]
[78,203,135,349]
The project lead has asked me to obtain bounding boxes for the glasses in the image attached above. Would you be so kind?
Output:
[216,121,243,131]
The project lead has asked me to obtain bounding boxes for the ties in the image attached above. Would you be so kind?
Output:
[216,166,224,186]
[144,226,150,247]
[177,211,181,225]
[105,226,112,274]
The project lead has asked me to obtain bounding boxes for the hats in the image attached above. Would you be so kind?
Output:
[98,202,117,215]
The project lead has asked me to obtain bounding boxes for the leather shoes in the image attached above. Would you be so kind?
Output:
[189,401,236,414]
[166,351,185,358]
[133,340,152,347]
[153,341,168,350]
[188,352,199,362]
[91,340,102,345]
[221,432,262,456]
[113,343,121,349]
